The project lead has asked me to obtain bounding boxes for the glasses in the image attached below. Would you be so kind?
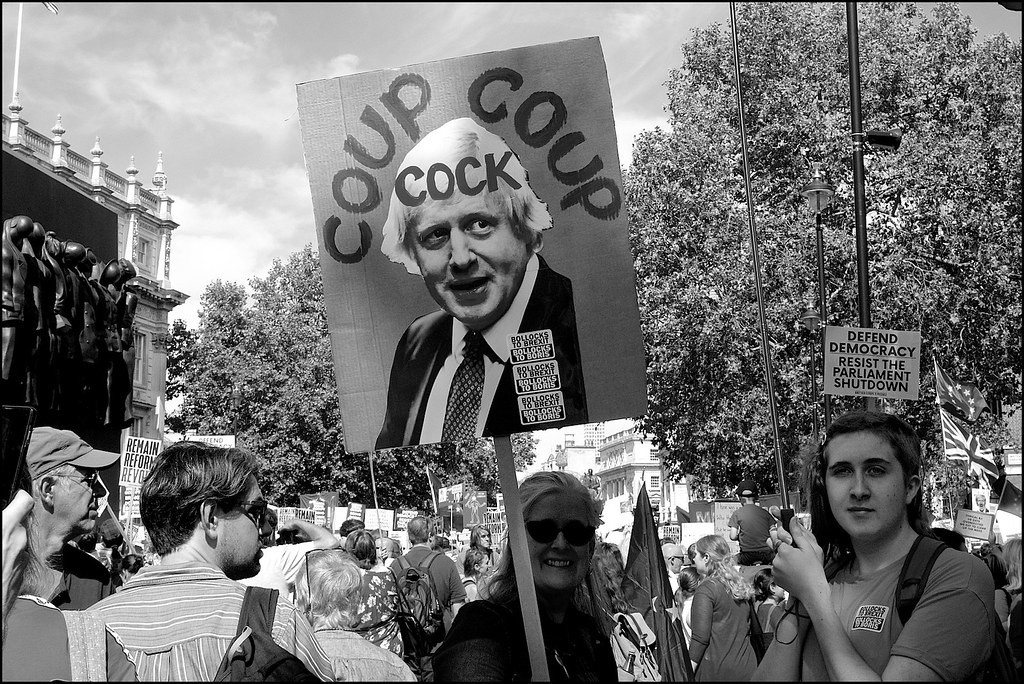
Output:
[525,521,597,547]
[668,556,684,563]
[217,498,266,527]
[305,547,348,603]
[51,471,99,489]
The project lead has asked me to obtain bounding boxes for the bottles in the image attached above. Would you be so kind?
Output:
[622,648,636,675]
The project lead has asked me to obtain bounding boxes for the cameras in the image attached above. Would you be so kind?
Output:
[279,530,304,545]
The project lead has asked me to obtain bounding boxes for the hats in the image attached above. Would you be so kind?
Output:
[18,426,122,481]
[737,480,758,497]
[100,519,124,542]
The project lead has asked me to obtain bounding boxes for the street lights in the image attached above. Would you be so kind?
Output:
[842,2,900,409]
[798,304,821,434]
[799,177,837,430]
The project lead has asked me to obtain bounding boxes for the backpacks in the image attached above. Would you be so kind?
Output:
[823,533,1020,684]
[213,586,321,684]
[580,584,662,683]
[395,551,444,639]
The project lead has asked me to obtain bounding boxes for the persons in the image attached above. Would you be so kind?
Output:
[431,535,456,554]
[0,488,36,624]
[375,116,590,452]
[930,528,1024,684]
[688,535,758,682]
[77,515,143,593]
[26,426,115,611]
[432,471,619,683]
[580,542,624,636]
[728,488,777,566]
[293,548,418,682]
[235,508,339,601]
[1,457,139,683]
[753,568,787,634]
[84,440,336,684]
[456,525,501,578]
[674,567,706,651]
[583,469,600,494]
[388,516,470,683]
[975,494,989,513]
[460,548,491,601]
[688,543,696,567]
[749,410,996,683]
[661,543,684,594]
[339,518,405,661]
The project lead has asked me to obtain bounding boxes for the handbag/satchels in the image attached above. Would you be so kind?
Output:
[748,596,766,668]
[386,567,429,659]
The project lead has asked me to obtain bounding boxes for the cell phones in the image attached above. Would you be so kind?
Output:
[0,405,38,511]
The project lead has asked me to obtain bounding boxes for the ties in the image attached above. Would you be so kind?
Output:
[441,333,485,444]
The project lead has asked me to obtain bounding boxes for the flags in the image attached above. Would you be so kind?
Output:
[621,482,695,681]
